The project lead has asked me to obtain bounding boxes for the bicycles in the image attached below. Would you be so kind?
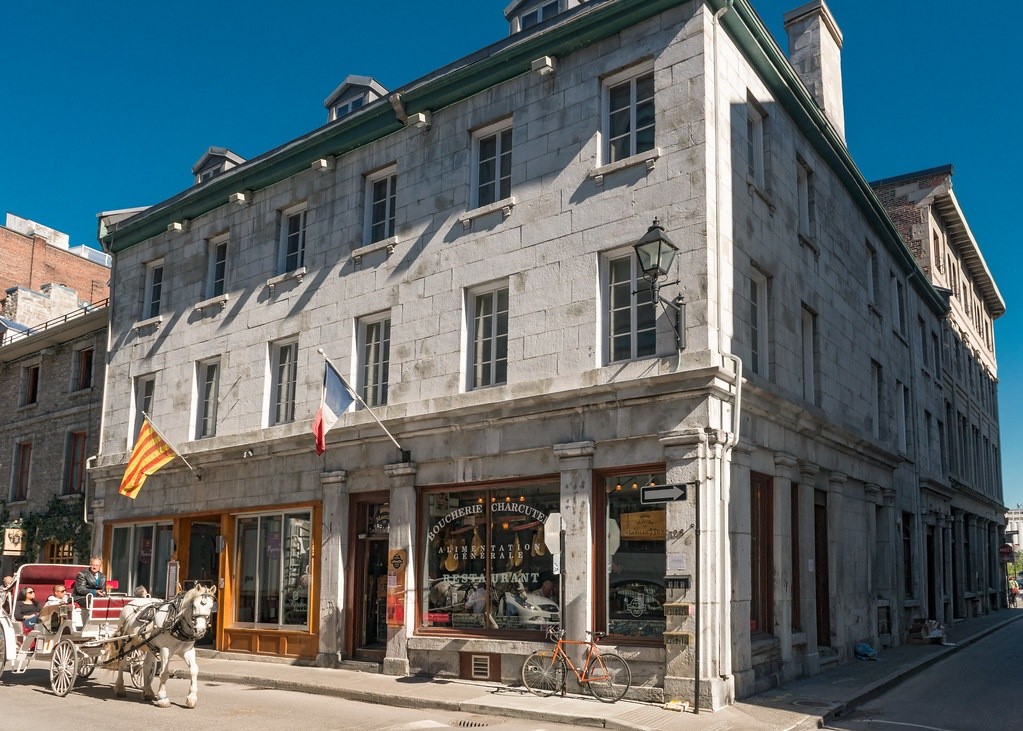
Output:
[1008,590,1018,609]
[521,625,631,703]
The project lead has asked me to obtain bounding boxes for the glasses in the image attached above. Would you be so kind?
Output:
[57,589,66,593]
[27,591,34,594]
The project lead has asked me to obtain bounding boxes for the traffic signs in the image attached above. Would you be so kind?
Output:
[999,544,1012,556]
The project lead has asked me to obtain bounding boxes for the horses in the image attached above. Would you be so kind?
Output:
[430,569,528,629]
[105,583,216,708]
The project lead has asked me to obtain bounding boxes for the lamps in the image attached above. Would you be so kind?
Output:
[531,55,559,78]
[477,491,483,504]
[629,215,686,351]
[357,528,367,543]
[406,110,432,135]
[504,490,511,503]
[519,489,525,502]
[491,490,496,503]
[167,218,191,236]
[616,477,622,492]
[632,476,638,491]
[311,154,336,176]
[650,475,655,486]
[229,189,254,205]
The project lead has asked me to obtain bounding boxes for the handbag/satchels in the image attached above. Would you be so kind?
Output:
[24,616,37,627]
[1011,587,1019,594]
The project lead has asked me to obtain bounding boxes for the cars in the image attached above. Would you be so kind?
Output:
[610,589,662,617]
[497,592,559,629]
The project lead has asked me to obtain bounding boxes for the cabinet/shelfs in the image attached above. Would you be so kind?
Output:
[376,577,387,642]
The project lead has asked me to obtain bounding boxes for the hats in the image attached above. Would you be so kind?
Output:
[1,570,12,578]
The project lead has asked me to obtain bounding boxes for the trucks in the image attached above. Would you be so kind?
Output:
[238,518,312,620]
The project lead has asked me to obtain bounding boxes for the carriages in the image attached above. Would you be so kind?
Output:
[0,564,218,710]
[428,569,527,628]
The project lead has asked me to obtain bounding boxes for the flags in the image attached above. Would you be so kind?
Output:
[119,421,175,499]
[312,362,353,456]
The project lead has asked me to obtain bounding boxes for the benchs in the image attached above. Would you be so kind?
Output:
[12,584,57,651]
[61,580,119,627]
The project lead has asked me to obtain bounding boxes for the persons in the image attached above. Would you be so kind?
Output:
[1009,576,1019,604]
[43,584,69,608]
[135,585,147,597]
[3,576,12,587]
[531,580,554,597]
[14,586,43,628]
[72,556,107,607]
[465,583,487,614]
